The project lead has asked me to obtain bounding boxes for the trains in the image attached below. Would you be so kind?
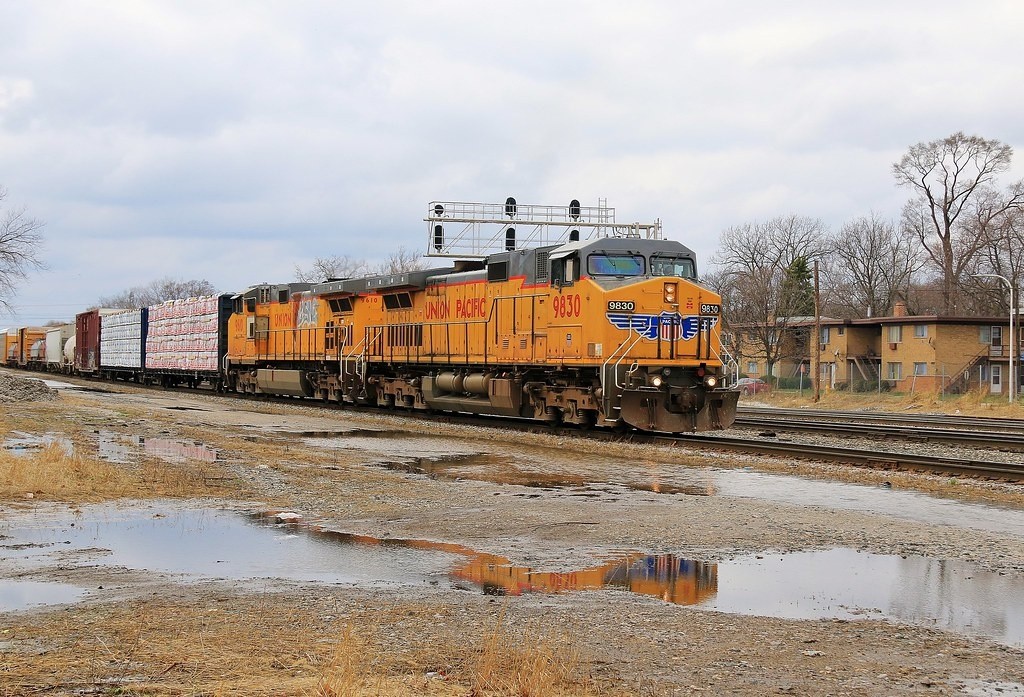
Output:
[0,237,742,434]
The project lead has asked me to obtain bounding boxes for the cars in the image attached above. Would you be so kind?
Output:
[729,378,771,396]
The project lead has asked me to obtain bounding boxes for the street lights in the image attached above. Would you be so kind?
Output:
[969,273,1014,404]
